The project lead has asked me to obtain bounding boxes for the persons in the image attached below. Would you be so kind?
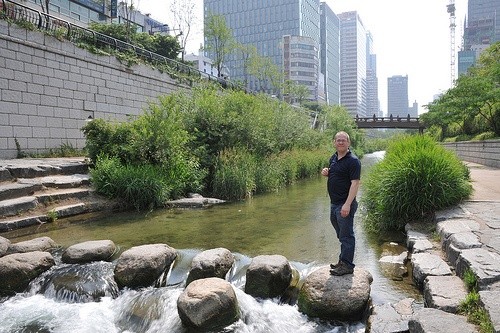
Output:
[320,131,361,277]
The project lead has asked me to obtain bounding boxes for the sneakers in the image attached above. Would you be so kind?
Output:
[330,264,354,276]
[331,261,344,268]
